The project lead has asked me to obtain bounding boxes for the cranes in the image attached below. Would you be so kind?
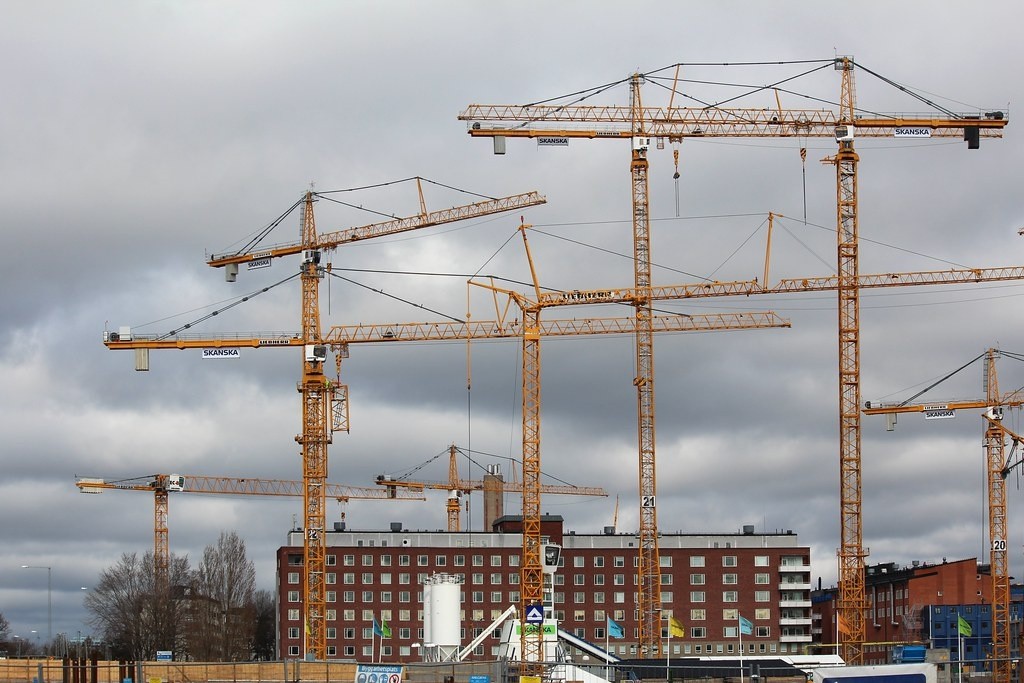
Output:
[72,53,1024,683]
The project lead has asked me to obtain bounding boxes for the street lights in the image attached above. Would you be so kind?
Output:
[21,565,52,658]
[30,630,40,652]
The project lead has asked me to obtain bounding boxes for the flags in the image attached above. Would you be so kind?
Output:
[383,620,392,637]
[306,620,312,635]
[838,614,850,635]
[608,618,623,639]
[740,616,753,635]
[373,618,383,636]
[670,616,684,637]
[959,616,971,636]
